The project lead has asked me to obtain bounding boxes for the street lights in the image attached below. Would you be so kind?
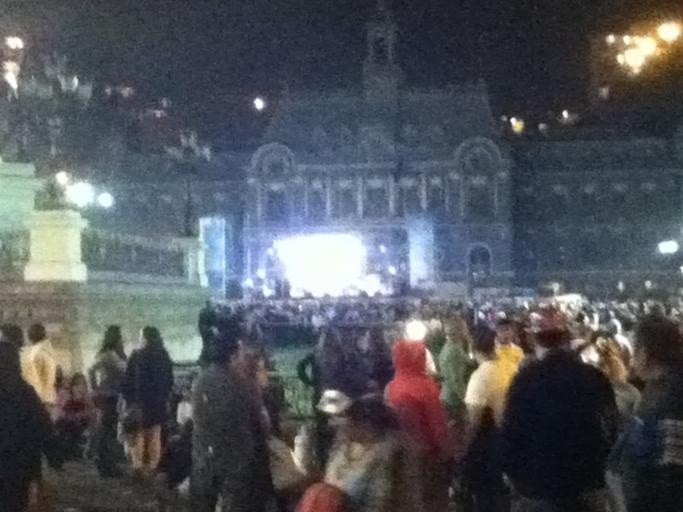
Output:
[163,125,211,241]
[13,45,104,211]
